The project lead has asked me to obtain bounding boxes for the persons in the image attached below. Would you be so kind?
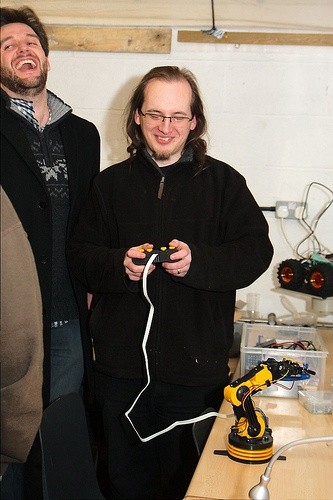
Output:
[74,66,273,500]
[0,6,100,500]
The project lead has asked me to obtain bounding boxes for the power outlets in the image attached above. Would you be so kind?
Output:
[275,201,308,220]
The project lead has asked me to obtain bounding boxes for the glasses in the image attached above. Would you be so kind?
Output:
[138,107,195,127]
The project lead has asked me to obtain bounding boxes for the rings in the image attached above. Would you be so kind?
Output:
[178,269,181,274]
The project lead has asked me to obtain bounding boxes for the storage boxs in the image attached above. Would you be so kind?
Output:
[240,323,333,415]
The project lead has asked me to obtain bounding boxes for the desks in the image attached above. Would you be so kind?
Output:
[182,326,333,500]
